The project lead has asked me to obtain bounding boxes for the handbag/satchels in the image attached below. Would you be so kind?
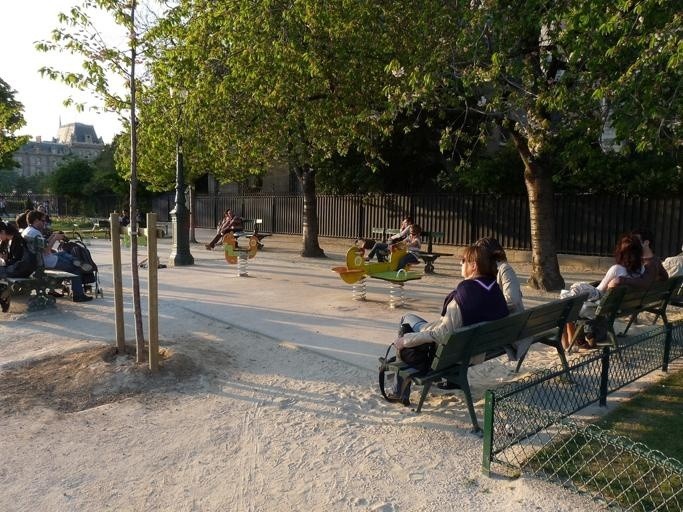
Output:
[5,243,33,278]
[398,322,437,374]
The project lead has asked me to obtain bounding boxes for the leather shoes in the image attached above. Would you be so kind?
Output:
[48,292,64,297]
[73,295,93,302]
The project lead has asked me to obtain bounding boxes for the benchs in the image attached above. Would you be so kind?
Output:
[368,227,454,274]
[4,233,79,313]
[232,217,271,250]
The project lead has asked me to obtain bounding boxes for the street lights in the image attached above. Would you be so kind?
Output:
[167,85,196,267]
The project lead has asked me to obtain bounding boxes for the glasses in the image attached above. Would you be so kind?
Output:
[460,258,469,263]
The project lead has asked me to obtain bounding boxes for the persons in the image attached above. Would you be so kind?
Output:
[660,244,682,279]
[386,245,506,399]
[560,235,644,352]
[206,202,428,285]
[0,192,160,319]
[470,235,526,319]
[592,226,670,291]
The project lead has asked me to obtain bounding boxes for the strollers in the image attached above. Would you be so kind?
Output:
[56,231,103,299]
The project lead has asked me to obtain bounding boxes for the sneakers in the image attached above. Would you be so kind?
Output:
[437,382,462,389]
[571,345,579,353]
[384,387,402,400]
[585,336,595,347]
[576,341,589,349]
[546,347,570,355]
